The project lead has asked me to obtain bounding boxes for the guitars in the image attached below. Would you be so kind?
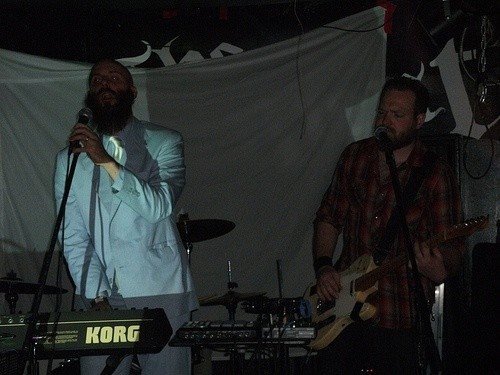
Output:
[299,217,488,351]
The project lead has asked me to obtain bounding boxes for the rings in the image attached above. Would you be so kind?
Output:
[84,136,90,143]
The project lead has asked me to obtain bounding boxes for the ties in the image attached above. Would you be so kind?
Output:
[108,136,124,164]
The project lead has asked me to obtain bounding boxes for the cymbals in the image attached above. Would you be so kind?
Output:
[176,220,237,243]
[0,277,69,294]
[201,291,267,306]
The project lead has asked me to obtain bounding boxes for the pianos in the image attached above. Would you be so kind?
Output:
[0,308,173,375]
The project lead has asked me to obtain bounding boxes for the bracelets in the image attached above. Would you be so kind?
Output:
[313,257,333,271]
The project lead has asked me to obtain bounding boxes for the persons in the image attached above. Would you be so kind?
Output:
[315,76,464,375]
[55,60,201,375]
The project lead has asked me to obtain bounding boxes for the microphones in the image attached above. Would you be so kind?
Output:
[68,107,94,156]
[374,125,393,144]
